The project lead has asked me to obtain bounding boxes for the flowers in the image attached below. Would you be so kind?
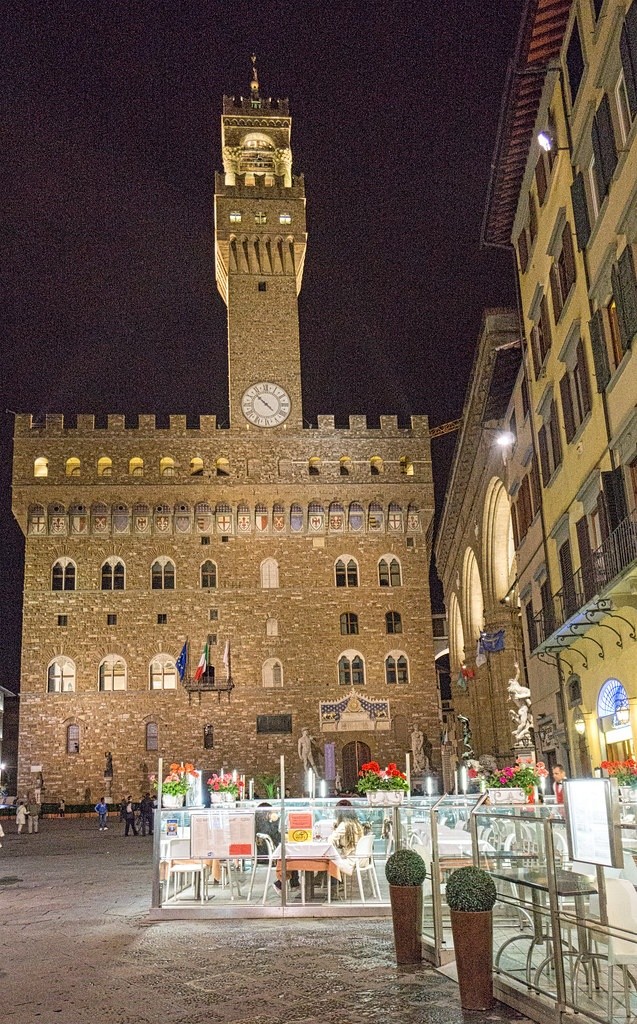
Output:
[150,762,200,797]
[468,757,551,795]
[355,762,412,793]
[208,772,244,796]
[601,759,637,788]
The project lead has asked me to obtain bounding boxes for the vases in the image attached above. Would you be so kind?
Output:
[163,793,186,809]
[210,791,238,809]
[487,787,530,807]
[620,786,637,804]
[365,788,406,808]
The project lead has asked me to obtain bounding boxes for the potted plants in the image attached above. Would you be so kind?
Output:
[386,849,427,965]
[164,805,637,904]
[447,866,497,1012]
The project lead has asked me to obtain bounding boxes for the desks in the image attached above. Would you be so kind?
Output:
[485,866,637,1018]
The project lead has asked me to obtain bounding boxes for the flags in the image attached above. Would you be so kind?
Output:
[193,639,209,683]
[223,639,229,683]
[175,638,188,682]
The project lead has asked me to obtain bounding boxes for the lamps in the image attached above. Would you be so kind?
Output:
[575,712,592,735]
[538,130,569,154]
[616,696,637,726]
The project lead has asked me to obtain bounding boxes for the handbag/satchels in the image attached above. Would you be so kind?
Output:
[15,815,19,824]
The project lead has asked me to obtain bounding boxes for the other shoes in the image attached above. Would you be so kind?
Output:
[99,828,102,831]
[272,884,282,898]
[104,827,108,830]
[125,834,129,836]
[134,833,139,836]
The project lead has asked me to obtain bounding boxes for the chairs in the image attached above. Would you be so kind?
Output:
[604,878,637,1024]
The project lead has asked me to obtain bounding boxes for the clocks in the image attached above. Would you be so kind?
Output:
[241,382,293,428]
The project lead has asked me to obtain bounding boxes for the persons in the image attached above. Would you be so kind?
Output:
[243,789,260,799]
[105,751,112,770]
[15,801,30,835]
[329,789,365,798]
[256,802,301,899]
[505,660,534,739]
[34,771,44,804]
[285,788,293,799]
[524,795,550,823]
[27,798,40,834]
[410,723,431,772]
[477,795,494,829]
[95,797,109,831]
[298,727,324,780]
[58,800,65,819]
[457,713,474,754]
[411,788,424,796]
[118,792,158,837]
[512,763,520,768]
[312,799,370,898]
[551,764,568,804]
